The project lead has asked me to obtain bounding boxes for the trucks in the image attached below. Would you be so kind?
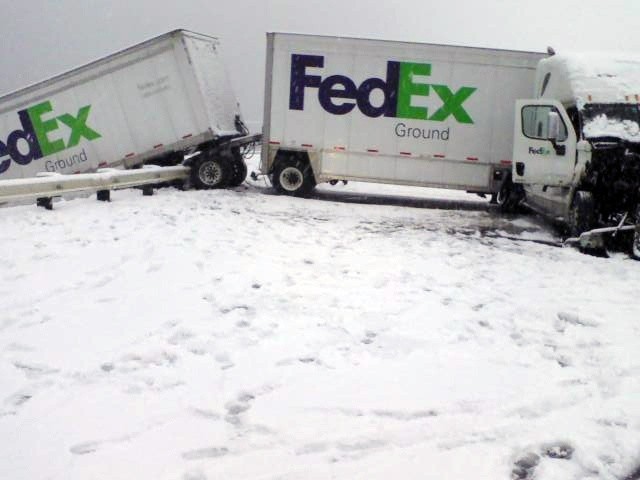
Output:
[1,27,638,260]
